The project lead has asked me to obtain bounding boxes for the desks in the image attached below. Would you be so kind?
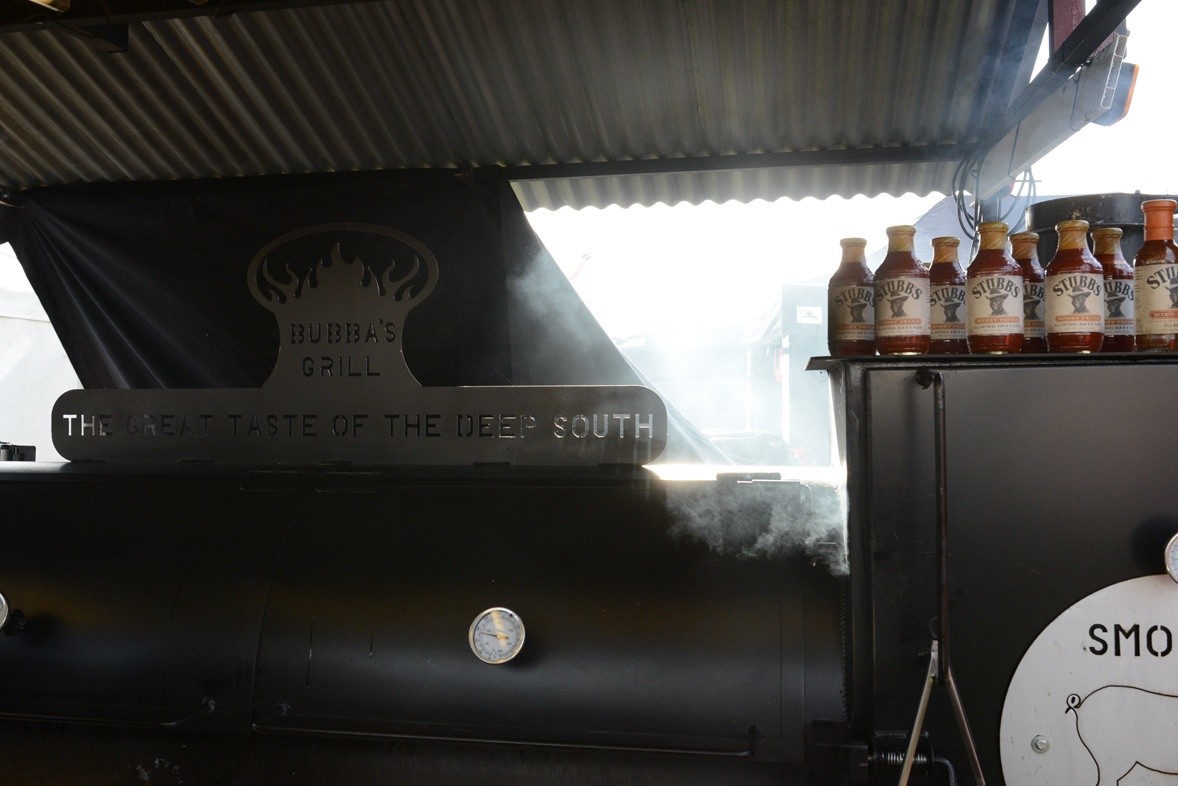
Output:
[804,352,1178,786]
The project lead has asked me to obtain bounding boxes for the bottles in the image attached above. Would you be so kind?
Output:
[1090,227,1138,355]
[1009,231,1050,353]
[965,220,1024,353]
[1043,220,1105,353]
[827,238,876,356]
[929,237,969,356]
[1133,199,1178,353]
[874,225,932,356]
